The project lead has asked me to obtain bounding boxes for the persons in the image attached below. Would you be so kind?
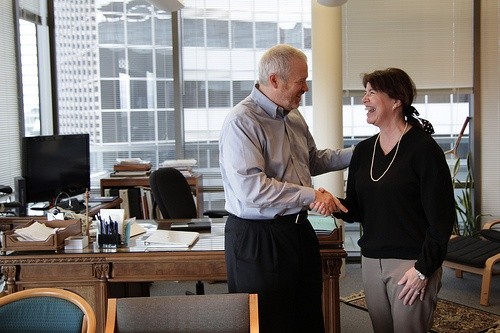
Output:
[220,44,357,333]
[310,68,456,333]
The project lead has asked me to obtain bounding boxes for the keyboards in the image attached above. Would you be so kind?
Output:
[57,198,90,213]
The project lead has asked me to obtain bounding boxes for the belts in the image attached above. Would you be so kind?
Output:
[248,211,307,226]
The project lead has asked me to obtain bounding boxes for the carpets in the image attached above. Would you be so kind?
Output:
[340,288,500,333]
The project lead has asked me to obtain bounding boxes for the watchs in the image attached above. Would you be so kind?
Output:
[413,266,429,281]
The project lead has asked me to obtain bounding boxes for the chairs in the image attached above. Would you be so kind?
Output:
[103,292,259,333]
[448,219,500,308]
[0,287,97,333]
[150,167,205,295]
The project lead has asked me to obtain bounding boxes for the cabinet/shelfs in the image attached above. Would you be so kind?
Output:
[0,216,347,333]
[99,170,203,217]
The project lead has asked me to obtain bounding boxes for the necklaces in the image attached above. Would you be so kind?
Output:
[370,120,408,182]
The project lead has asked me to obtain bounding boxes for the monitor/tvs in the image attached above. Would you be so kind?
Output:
[21,134,90,204]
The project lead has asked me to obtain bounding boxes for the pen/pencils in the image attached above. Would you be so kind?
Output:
[94,213,118,236]
[172,222,196,225]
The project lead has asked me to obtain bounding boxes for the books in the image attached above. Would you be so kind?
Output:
[110,158,198,177]
[145,230,199,248]
[105,185,197,220]
